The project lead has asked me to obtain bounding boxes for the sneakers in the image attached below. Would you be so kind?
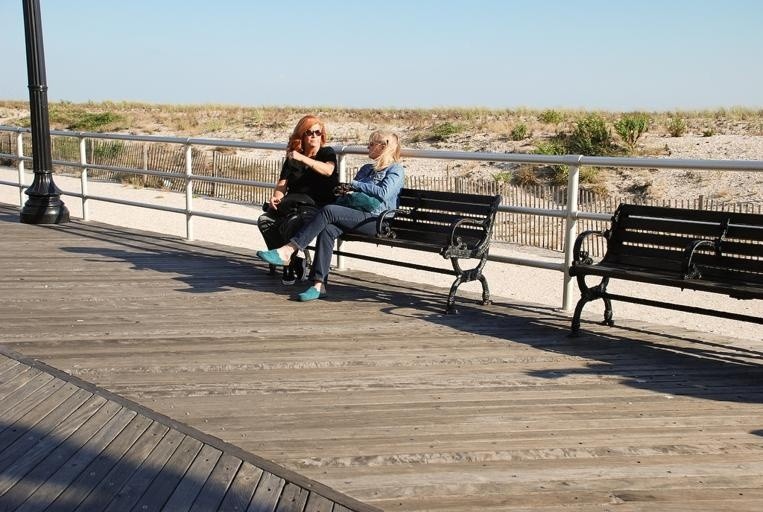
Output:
[281,256,307,285]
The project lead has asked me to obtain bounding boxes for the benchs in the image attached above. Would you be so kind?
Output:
[333,182,503,317]
[565,203,763,336]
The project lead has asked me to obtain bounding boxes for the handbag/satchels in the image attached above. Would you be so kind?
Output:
[336,191,380,211]
[275,193,314,211]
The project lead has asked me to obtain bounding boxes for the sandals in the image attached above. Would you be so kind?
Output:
[257,249,290,266]
[298,286,328,302]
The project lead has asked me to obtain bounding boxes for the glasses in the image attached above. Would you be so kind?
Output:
[369,142,383,146]
[304,129,321,136]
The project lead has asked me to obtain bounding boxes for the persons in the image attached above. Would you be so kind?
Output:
[258,115,339,286]
[256,130,405,302]
